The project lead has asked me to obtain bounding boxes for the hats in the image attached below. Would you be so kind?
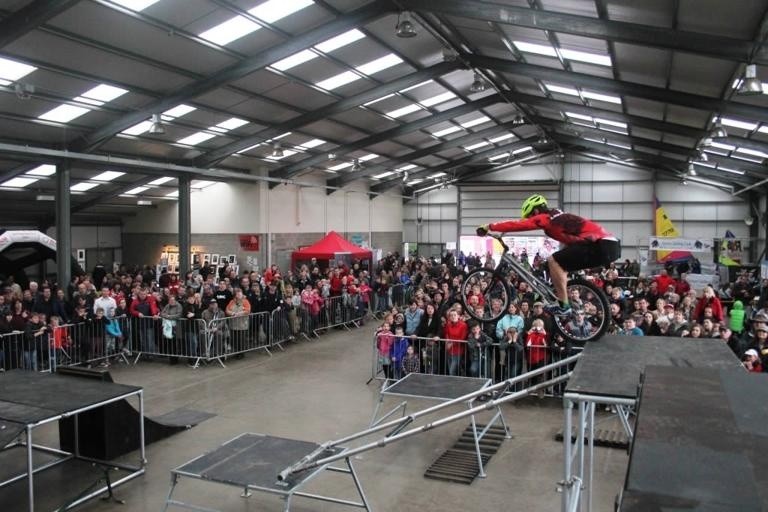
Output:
[754,326,768,333]
[748,312,768,323]
[744,348,759,356]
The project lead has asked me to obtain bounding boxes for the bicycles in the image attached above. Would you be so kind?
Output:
[460,228,612,344]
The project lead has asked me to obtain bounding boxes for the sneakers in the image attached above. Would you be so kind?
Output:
[662,302,734,337]
[542,304,573,318]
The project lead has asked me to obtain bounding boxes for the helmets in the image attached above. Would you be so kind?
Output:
[520,193,548,218]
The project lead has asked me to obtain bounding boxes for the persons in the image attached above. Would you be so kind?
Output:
[1,249,768,399]
[476,194,621,316]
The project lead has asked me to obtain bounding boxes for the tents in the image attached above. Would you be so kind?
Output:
[292,232,374,278]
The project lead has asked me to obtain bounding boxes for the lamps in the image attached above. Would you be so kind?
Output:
[271,141,284,158]
[736,63,763,98]
[679,113,729,187]
[147,113,167,135]
[350,159,365,173]
[402,170,412,182]
[395,11,418,39]
[468,71,549,147]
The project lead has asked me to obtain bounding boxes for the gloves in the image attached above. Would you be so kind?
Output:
[475,223,490,237]
